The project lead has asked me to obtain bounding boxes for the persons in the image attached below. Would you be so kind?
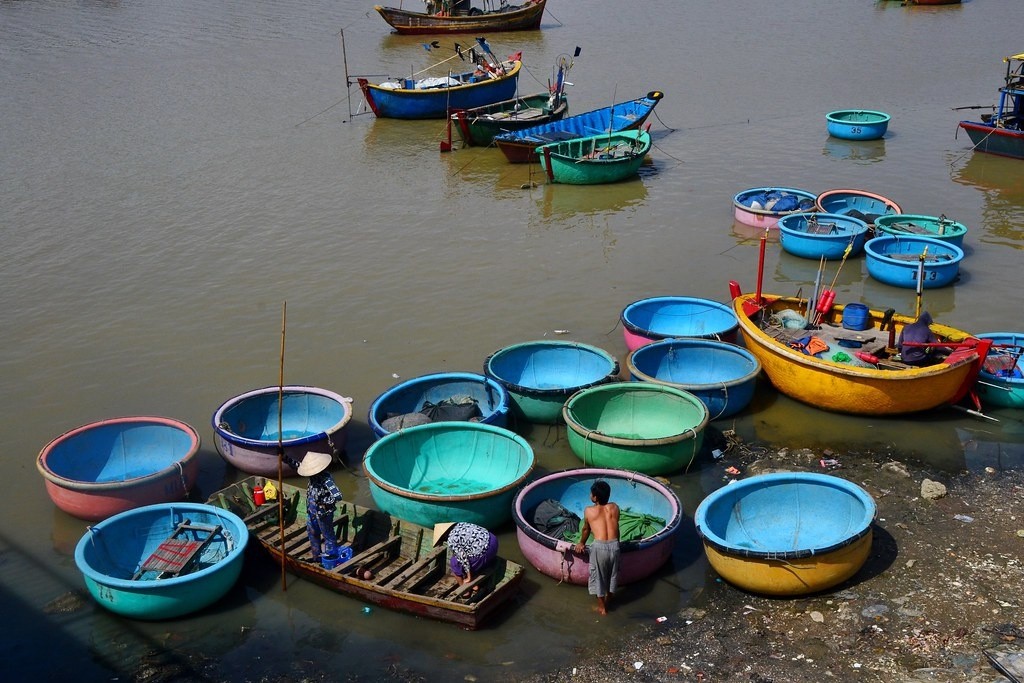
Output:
[277,447,342,562]
[574,481,620,616]
[442,0,449,17]
[898,312,952,367]
[433,522,498,598]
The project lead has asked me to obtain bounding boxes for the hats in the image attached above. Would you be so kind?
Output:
[297,450,332,477]
[432,522,459,548]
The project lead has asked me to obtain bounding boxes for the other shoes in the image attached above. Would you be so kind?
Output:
[306,557,321,563]
[328,554,337,559]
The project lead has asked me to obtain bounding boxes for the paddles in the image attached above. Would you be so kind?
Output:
[278,300,288,592]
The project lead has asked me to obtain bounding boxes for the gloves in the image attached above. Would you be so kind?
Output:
[276,445,285,456]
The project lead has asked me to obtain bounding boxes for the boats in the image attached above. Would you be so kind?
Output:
[625,336,764,422]
[863,235,965,290]
[776,212,869,262]
[562,380,710,477]
[483,338,620,426]
[815,188,902,242]
[618,295,740,356]
[872,213,968,253]
[825,109,892,142]
[450,46,582,149]
[729,222,993,418]
[490,90,664,165]
[205,473,527,631]
[954,54,1024,161]
[373,0,547,36]
[970,331,1024,410]
[356,36,523,119]
[533,122,652,185]
[210,383,355,481]
[691,468,878,599]
[510,466,685,589]
[731,187,819,230]
[35,416,201,523]
[367,369,511,445]
[74,500,250,622]
[361,420,539,536]
